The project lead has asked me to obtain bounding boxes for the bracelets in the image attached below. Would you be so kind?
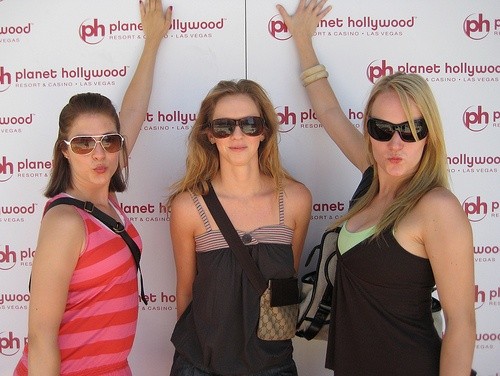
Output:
[299,64,330,87]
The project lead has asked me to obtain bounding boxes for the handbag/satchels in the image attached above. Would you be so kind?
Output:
[300,226,339,342]
[254,276,300,342]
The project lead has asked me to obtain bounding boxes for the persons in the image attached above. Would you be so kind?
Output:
[169,78,312,376]
[276,1,479,376]
[11,1,173,376]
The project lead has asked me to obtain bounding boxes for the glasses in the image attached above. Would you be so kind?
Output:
[63,133,125,156]
[365,114,430,143]
[207,115,267,139]
